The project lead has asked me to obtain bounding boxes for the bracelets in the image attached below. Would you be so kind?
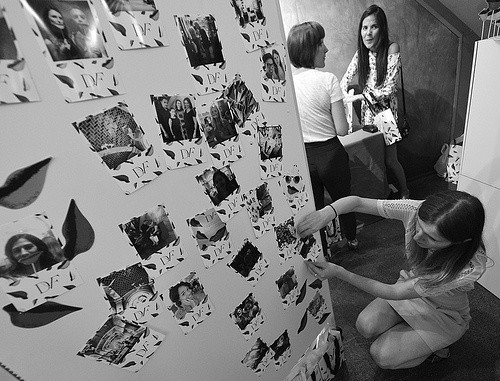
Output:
[328,204,339,220]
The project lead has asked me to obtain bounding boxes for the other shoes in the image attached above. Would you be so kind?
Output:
[401,189,409,199]
[425,348,451,364]
[347,238,359,250]
[388,188,401,199]
[324,254,330,263]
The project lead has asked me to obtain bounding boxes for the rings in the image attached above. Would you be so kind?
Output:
[314,272,317,278]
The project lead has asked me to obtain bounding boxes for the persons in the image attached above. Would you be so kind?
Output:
[0,0,332,337]
[285,22,359,260]
[298,190,496,372]
[340,4,411,201]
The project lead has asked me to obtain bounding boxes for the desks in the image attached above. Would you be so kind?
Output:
[336,124,388,229]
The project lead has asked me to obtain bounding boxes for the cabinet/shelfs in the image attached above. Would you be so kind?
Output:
[457,36,500,299]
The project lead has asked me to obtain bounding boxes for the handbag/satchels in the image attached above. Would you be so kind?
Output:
[398,117,410,140]
[434,138,463,185]
[362,92,402,147]
